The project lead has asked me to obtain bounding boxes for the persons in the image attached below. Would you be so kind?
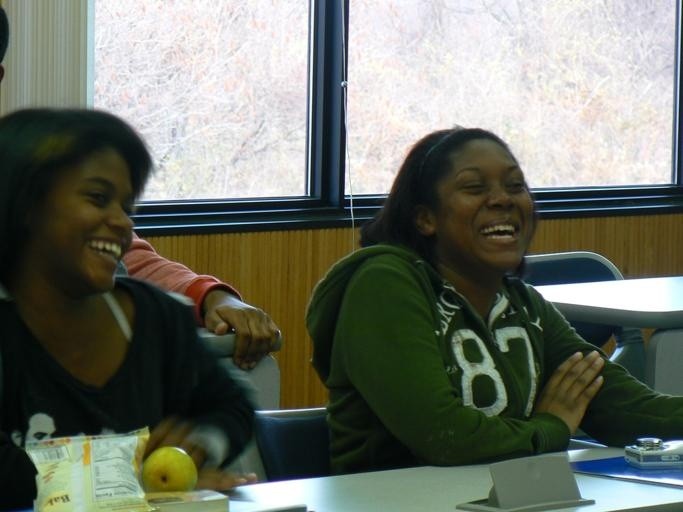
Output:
[2,109,258,512]
[2,8,276,370]
[305,127,683,474]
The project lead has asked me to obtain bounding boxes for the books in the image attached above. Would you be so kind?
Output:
[147,487,227,512]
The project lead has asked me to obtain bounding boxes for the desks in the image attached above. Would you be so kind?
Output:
[27,446,683,512]
[534,276,683,398]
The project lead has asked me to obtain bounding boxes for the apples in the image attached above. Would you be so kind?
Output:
[142,447,198,492]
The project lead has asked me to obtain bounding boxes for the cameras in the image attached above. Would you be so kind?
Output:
[624,438,683,470]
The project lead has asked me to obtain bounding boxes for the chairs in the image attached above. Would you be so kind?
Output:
[249,407,604,481]
[519,252,646,385]
[196,326,283,410]
